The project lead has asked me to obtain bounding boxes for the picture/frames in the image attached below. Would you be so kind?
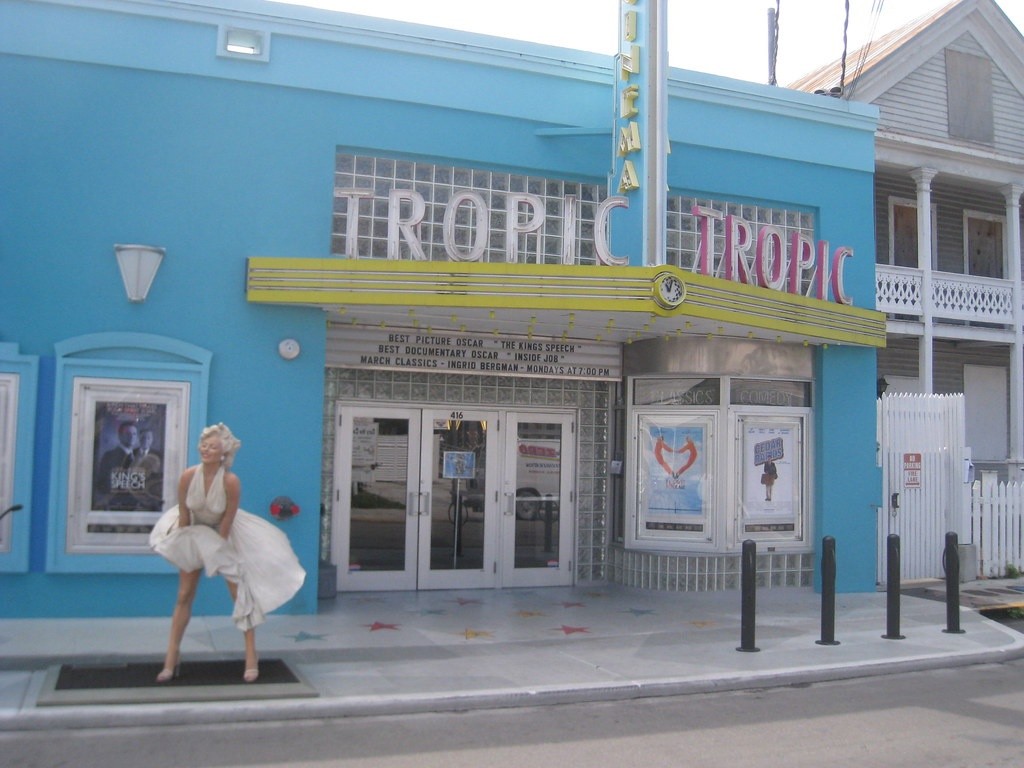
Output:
[64,375,192,555]
[737,420,803,543]
[0,371,20,554]
[636,414,717,543]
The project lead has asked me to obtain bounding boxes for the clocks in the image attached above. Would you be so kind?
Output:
[651,272,686,311]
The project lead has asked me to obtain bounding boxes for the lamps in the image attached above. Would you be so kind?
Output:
[215,26,271,62]
[114,244,166,304]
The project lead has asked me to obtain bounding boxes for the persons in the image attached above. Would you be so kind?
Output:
[121,425,162,511]
[763,455,778,501]
[139,421,306,686]
[93,420,141,511]
